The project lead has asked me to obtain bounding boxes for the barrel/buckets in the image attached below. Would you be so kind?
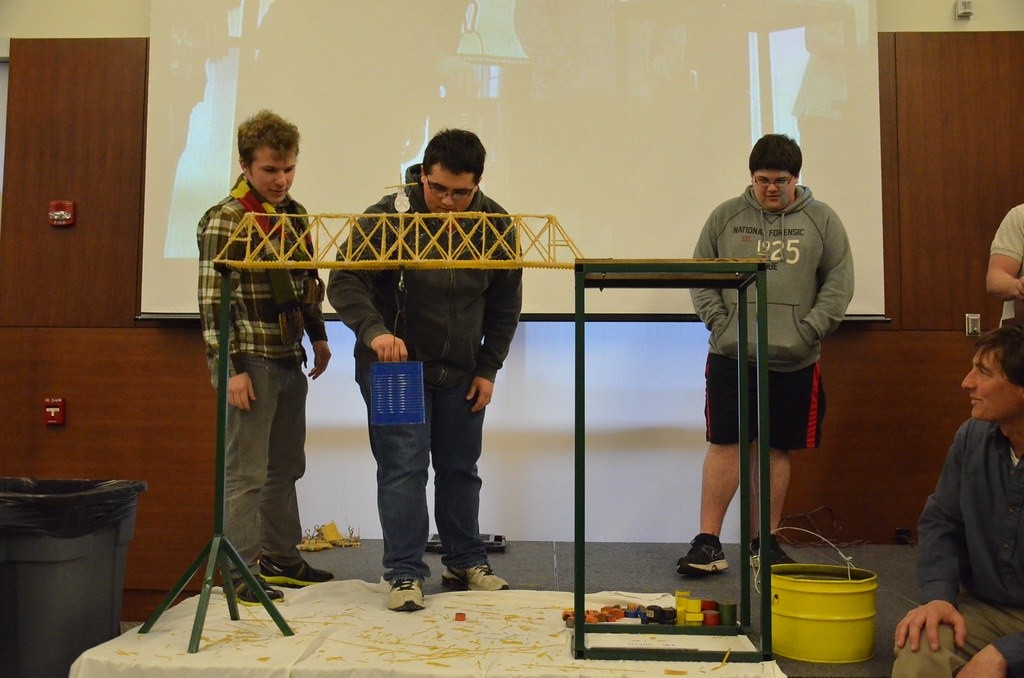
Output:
[751,526,877,663]
[370,362,426,427]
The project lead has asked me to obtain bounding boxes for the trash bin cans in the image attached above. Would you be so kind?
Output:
[0,478,148,678]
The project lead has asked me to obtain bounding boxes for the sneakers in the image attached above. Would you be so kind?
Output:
[677,534,729,575]
[259,556,334,588]
[388,578,425,612]
[749,534,797,569]
[442,564,510,591]
[222,575,284,606]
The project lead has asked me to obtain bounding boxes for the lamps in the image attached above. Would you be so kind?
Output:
[956,0,974,21]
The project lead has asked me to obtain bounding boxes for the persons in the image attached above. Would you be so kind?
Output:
[327,125,522,612]
[199,110,333,606]
[986,204,1024,331]
[892,327,1024,678]
[674,132,854,575]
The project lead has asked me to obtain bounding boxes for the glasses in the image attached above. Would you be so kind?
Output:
[753,173,793,188]
[426,175,478,201]
